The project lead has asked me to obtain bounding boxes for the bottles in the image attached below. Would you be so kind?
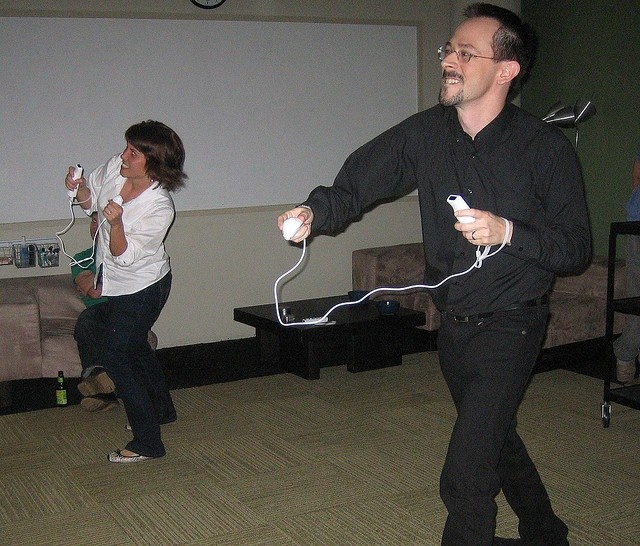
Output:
[55,371,67,407]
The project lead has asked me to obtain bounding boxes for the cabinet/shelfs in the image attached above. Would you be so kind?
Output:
[601,215,639,432]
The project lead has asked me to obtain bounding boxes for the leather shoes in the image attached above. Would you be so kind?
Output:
[106,450,160,464]
[78,365,116,398]
[80,399,119,415]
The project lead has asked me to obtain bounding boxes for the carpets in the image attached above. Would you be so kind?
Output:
[0,339,639,546]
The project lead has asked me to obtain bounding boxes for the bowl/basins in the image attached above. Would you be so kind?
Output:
[348,291,370,305]
[377,300,400,316]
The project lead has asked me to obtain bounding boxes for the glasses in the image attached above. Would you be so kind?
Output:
[438,44,521,63]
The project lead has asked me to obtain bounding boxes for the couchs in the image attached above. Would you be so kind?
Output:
[349,240,621,347]
[0,274,158,383]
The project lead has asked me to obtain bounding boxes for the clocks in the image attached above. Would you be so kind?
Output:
[190,0,230,8]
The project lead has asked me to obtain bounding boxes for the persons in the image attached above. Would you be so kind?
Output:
[73,211,158,414]
[65,120,190,465]
[277,1,594,546]
[614,151,640,384]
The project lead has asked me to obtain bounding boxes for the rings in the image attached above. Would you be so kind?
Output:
[472,231,477,240]
[108,211,111,215]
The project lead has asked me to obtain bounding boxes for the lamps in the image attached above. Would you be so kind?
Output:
[549,107,577,129]
[540,101,566,120]
[574,97,598,125]
